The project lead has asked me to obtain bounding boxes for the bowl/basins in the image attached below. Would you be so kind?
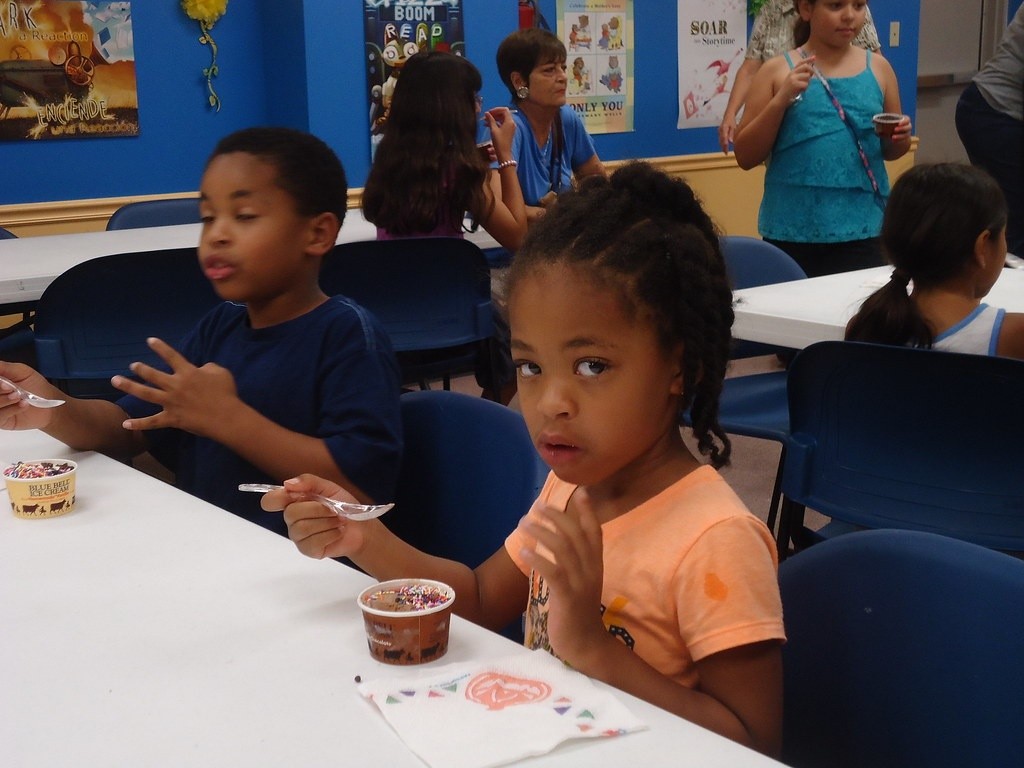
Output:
[873,112,904,139]
[478,144,491,153]
[358,578,456,666]
[3,459,77,519]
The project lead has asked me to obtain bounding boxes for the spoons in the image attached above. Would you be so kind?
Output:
[238,483,395,521]
[0,375,66,408]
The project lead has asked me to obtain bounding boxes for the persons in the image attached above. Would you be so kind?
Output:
[844,160,1024,361]
[479,29,608,220]
[0,126,403,565]
[358,50,530,406]
[955,0,1024,256]
[718,1,913,279]
[261,153,787,762]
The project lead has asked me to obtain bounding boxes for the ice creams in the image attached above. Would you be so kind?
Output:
[4,460,74,479]
[364,583,449,613]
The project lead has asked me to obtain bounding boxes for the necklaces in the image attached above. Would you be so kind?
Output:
[543,123,552,158]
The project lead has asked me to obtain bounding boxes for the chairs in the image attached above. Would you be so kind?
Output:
[0,226,40,367]
[318,235,1024,768]
[106,197,205,231]
[27,248,229,469]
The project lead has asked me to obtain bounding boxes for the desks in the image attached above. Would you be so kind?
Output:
[0,209,504,365]
[730,265,1024,562]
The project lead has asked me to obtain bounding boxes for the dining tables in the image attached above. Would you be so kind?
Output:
[0,425,794,768]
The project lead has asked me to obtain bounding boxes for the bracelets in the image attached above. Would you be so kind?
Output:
[498,160,517,171]
